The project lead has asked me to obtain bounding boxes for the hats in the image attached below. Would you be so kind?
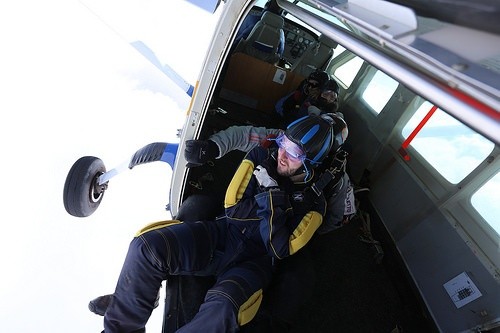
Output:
[309,71,331,85]
[275,113,334,169]
[319,80,340,95]
[326,112,348,153]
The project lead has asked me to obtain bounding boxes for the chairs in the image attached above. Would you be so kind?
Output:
[289,34,338,77]
[236,11,285,64]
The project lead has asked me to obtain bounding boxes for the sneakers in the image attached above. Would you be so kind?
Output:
[87,288,161,317]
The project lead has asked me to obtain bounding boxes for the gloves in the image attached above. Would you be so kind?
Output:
[181,139,220,168]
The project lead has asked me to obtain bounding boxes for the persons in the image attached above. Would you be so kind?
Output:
[236,0,360,120]
[88,113,359,333]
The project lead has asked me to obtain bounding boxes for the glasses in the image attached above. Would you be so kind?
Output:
[307,81,317,88]
